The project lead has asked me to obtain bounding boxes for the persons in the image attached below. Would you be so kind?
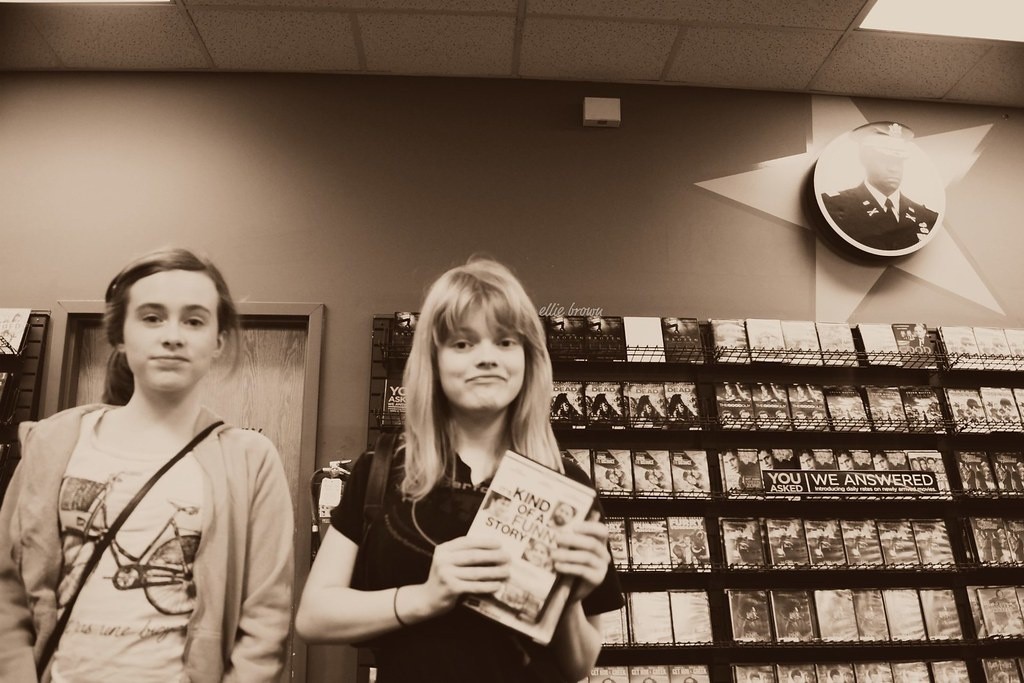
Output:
[820,122,939,250]
[2,314,20,346]
[548,503,577,532]
[529,543,551,568]
[0,250,295,683]
[483,493,511,518]
[398,312,410,328]
[295,257,622,683]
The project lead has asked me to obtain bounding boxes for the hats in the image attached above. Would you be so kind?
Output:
[851,120,916,160]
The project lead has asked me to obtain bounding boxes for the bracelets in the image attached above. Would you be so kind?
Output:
[394,587,408,627]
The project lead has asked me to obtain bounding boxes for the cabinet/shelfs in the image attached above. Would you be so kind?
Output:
[368,308,1024,683]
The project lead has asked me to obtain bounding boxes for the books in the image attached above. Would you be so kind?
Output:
[546,317,1024,683]
[463,511,601,644]
[456,446,597,625]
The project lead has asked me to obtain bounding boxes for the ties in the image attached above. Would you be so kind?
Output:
[920,340,924,349]
[883,198,898,227]
